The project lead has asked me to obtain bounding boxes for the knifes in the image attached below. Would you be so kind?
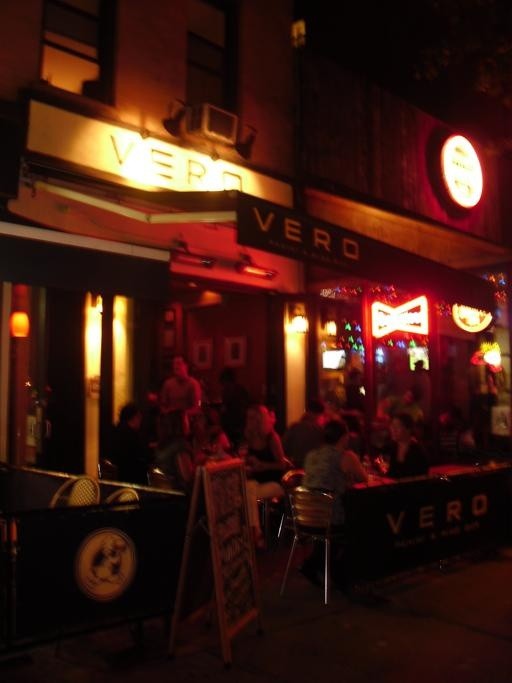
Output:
[192,98,242,145]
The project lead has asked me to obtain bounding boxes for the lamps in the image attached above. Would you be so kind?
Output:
[267,480,349,607]
[254,462,308,544]
[96,484,157,647]
[45,473,106,660]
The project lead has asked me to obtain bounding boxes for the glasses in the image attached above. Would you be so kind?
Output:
[236,121,257,161]
[163,96,188,137]
[235,252,277,280]
[166,240,214,267]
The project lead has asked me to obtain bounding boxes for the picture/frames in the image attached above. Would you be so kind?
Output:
[353,470,396,488]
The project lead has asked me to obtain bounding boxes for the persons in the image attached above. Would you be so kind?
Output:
[104,355,281,552]
[281,354,511,587]
